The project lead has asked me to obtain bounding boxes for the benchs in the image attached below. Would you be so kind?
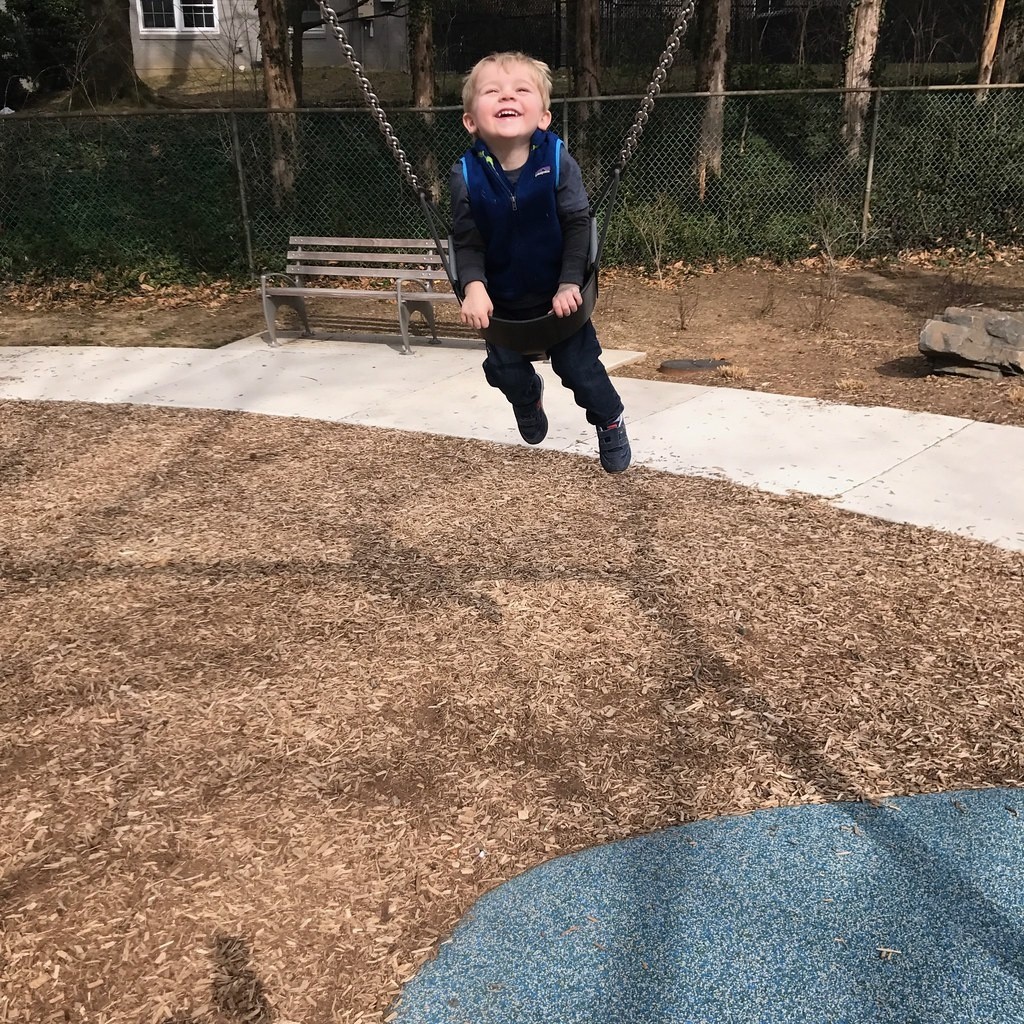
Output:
[261,236,458,355]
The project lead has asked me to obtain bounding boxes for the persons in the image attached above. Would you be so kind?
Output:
[450,52,631,474]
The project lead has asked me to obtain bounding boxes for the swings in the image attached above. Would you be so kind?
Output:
[315,0,696,362]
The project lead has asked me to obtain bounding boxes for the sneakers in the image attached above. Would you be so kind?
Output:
[513,373,548,445]
[597,413,631,474]
